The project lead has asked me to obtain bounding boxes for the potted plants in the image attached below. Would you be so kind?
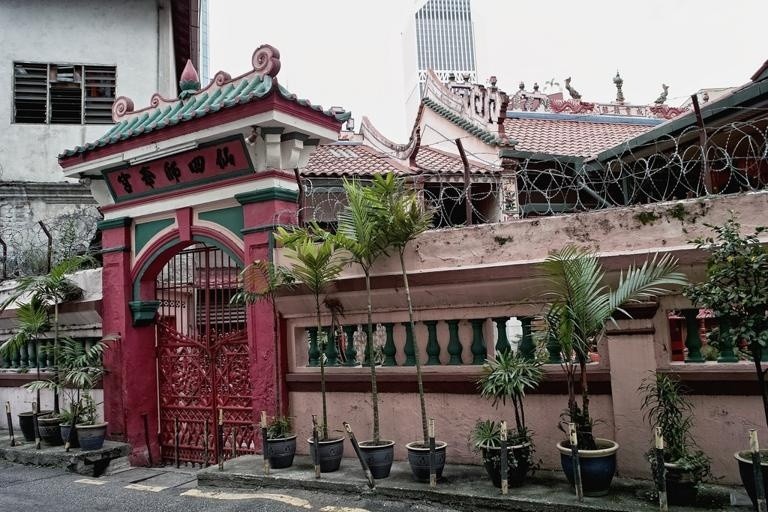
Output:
[229,259,298,470]
[370,168,449,483]
[680,209,768,512]
[271,217,346,474]
[521,244,688,504]
[466,348,547,489]
[332,174,396,479]
[637,368,707,505]
[1,244,121,446]
